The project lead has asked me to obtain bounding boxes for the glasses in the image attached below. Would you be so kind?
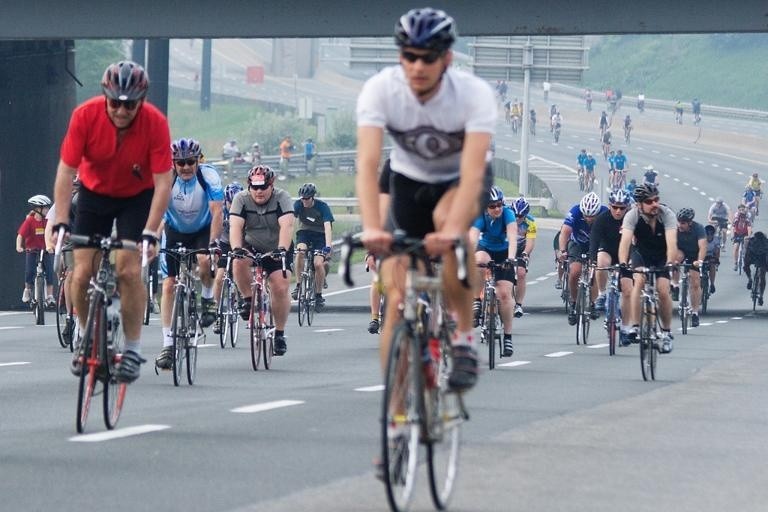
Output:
[109,98,142,111]
[487,202,503,209]
[399,50,444,64]
[22,288,31,303]
[251,184,271,191]
[301,198,312,200]
[44,296,58,309]
[174,159,197,167]
[611,205,627,210]
[642,197,659,205]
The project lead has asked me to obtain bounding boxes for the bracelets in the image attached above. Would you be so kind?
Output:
[142,229,159,237]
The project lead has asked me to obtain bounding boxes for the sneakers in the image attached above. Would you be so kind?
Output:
[472,298,483,318]
[447,345,479,389]
[62,286,325,385]
[367,320,380,334]
[513,304,524,317]
[370,439,409,487]
[502,338,514,358]
[567,279,765,353]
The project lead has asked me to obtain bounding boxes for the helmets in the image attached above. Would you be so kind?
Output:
[579,192,601,217]
[26,194,52,208]
[100,60,150,104]
[609,189,631,206]
[633,181,659,203]
[298,183,317,197]
[169,138,202,161]
[393,8,457,53]
[223,182,244,202]
[487,185,503,202]
[247,166,277,186]
[676,206,695,222]
[510,197,529,218]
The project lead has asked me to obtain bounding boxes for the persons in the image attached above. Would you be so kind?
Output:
[44,56,177,386]
[350,6,500,486]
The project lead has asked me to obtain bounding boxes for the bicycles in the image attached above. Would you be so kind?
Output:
[49,220,151,435]
[325,230,471,512]
